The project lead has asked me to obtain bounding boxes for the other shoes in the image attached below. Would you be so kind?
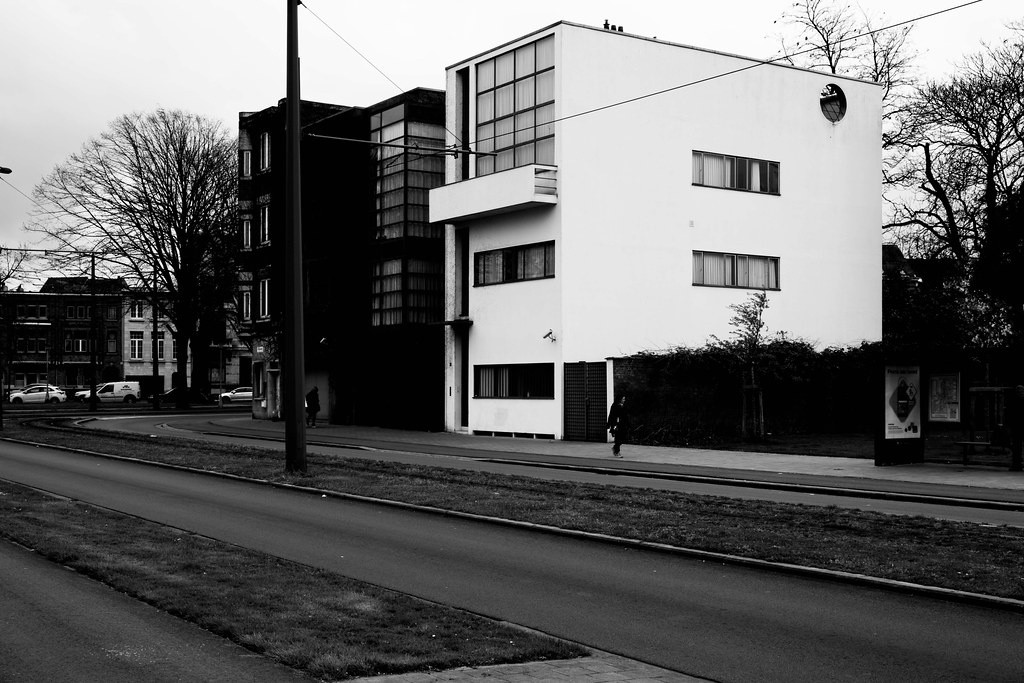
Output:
[616,453,623,458]
[612,448,616,455]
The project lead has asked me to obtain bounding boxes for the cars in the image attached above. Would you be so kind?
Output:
[7,383,61,396]
[7,386,67,405]
[149,386,210,406]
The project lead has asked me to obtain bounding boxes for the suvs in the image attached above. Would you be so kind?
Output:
[219,386,253,405]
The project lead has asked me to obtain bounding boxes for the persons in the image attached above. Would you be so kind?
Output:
[305,385,322,429]
[607,392,628,458]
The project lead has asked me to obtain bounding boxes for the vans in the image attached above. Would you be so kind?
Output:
[74,381,141,405]
